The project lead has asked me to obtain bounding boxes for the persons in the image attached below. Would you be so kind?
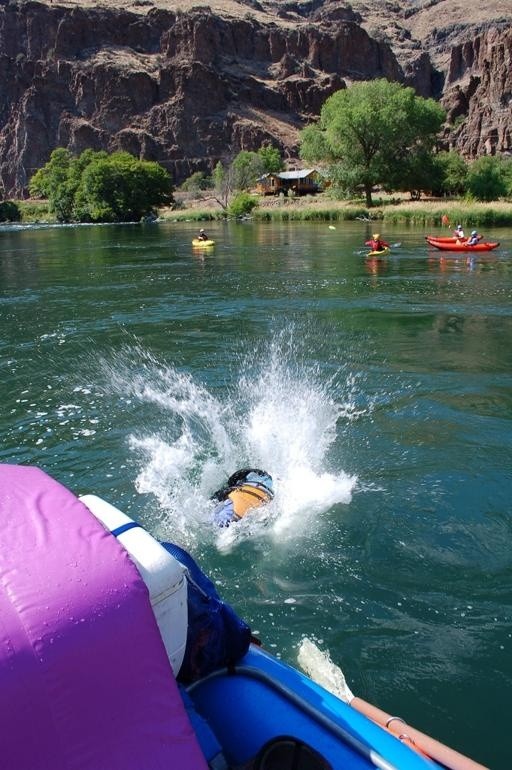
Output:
[466,230,479,246]
[468,255,477,273]
[448,224,464,244]
[198,228,207,240]
[365,232,388,253]
[366,257,388,276]
[195,250,208,262]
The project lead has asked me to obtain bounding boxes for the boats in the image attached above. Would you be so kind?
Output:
[189,238,217,247]
[365,247,393,257]
[0,459,495,770]
[191,246,216,254]
[423,234,501,252]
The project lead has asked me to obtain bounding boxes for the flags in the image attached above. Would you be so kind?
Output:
[442,214,448,225]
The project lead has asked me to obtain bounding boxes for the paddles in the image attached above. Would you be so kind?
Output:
[356,242,403,252]
[442,215,458,235]
[297,637,490,770]
